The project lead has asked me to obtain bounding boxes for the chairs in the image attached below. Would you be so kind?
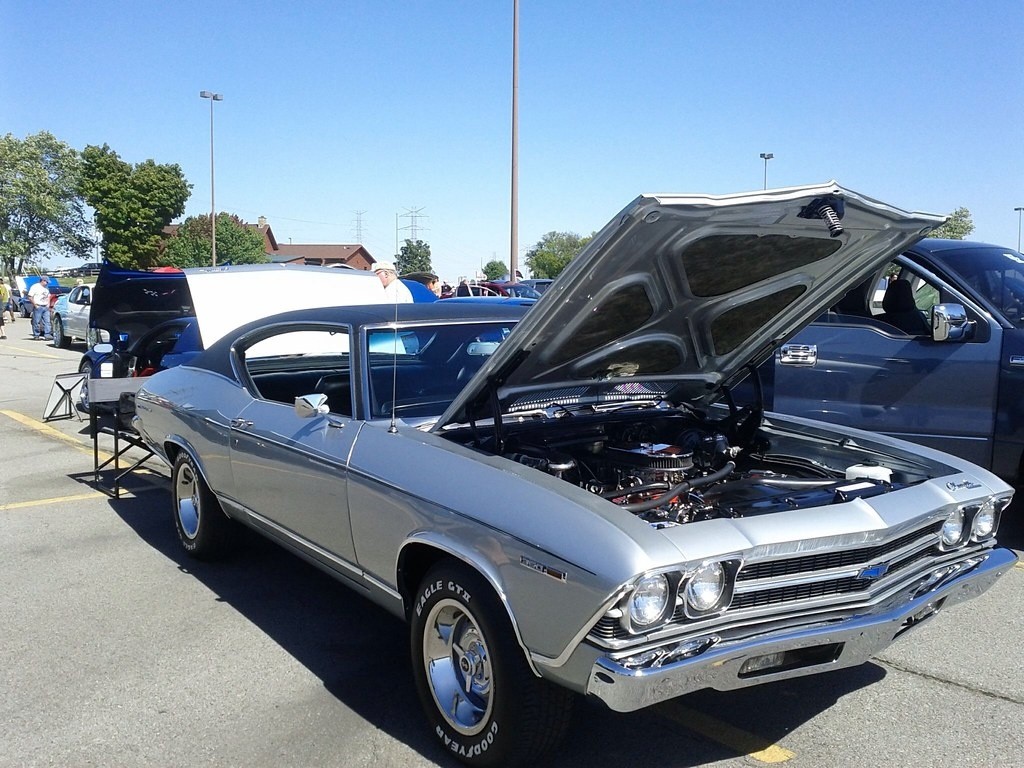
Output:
[314,373,385,420]
[880,278,933,335]
[828,271,874,318]
[455,355,490,395]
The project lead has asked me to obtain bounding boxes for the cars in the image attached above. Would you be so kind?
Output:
[11,276,73,318]
[78,264,205,415]
[131,179,1020,766]
[52,283,111,351]
[399,278,554,352]
[717,238,1024,480]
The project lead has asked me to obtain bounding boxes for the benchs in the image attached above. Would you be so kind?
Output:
[248,364,458,412]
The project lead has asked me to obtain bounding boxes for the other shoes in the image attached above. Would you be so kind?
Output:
[34,336,40,341]
[44,333,53,340]
[0,336,7,339]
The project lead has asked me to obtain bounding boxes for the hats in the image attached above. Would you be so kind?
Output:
[370,261,396,272]
[41,275,52,284]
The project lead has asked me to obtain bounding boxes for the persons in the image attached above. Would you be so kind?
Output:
[74,279,88,302]
[370,261,419,354]
[28,276,53,340]
[888,273,898,287]
[0,277,15,339]
[427,275,470,297]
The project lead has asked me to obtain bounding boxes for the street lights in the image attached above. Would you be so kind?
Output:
[200,91,223,267]
[1014,208,1024,254]
[760,153,774,191]
